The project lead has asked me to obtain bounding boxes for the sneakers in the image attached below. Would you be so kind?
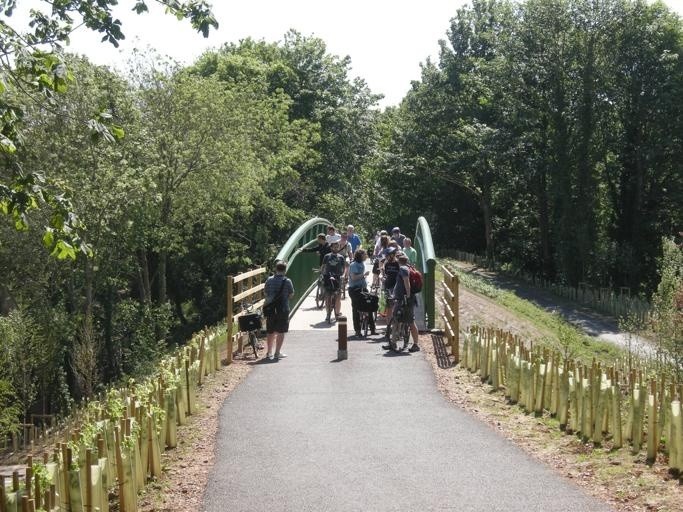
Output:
[325,312,343,324]
[266,352,287,359]
[370,328,379,335]
[382,342,397,350]
[355,330,362,338]
[408,345,420,351]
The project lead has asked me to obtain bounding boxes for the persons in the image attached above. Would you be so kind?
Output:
[299,224,423,352]
[263,261,295,359]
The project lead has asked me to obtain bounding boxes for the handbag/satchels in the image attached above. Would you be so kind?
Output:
[324,274,340,293]
[238,314,262,332]
[393,279,404,300]
[263,299,281,317]
[352,291,379,312]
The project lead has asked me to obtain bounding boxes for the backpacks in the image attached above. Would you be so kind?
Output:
[405,264,423,293]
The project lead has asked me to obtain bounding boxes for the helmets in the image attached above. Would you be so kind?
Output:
[385,247,395,256]
[392,226,400,233]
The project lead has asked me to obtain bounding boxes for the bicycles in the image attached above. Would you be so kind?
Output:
[309,256,411,352]
